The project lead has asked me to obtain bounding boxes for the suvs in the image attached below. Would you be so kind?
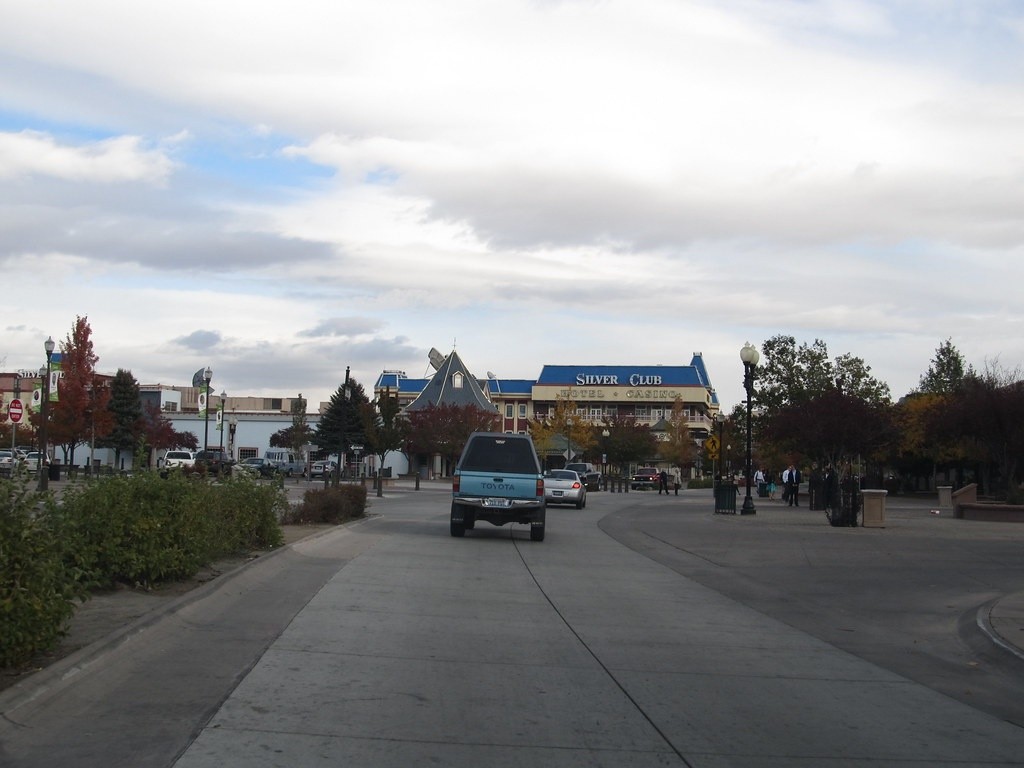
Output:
[631,467,660,490]
[160,451,198,479]
[563,463,601,492]
[194,448,236,476]
[450,432,546,539]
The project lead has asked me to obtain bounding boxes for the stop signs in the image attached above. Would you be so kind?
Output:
[8,399,24,423]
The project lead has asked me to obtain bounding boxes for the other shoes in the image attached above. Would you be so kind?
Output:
[788,504,792,506]
[796,504,798,506]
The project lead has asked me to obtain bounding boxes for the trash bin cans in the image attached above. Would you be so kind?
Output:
[937,487,953,507]
[48,465,61,481]
[759,481,770,497]
[860,489,888,527]
[714,484,738,515]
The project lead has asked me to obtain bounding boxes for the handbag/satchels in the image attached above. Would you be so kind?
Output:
[755,472,758,482]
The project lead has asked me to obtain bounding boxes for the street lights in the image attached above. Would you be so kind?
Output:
[36,335,55,492]
[220,390,227,453]
[229,417,238,458]
[205,366,213,452]
[34,364,47,479]
[739,341,759,515]
[602,429,610,491]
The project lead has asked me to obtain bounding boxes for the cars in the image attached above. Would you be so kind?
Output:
[0,451,20,475]
[231,457,275,480]
[274,460,294,476]
[14,446,39,458]
[542,469,588,508]
[305,461,337,480]
[23,452,50,474]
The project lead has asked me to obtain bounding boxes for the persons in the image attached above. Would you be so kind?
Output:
[658,468,670,495]
[672,469,681,496]
[753,466,765,494]
[782,465,792,503]
[715,471,722,480]
[764,468,775,501]
[788,463,801,507]
[733,471,741,495]
